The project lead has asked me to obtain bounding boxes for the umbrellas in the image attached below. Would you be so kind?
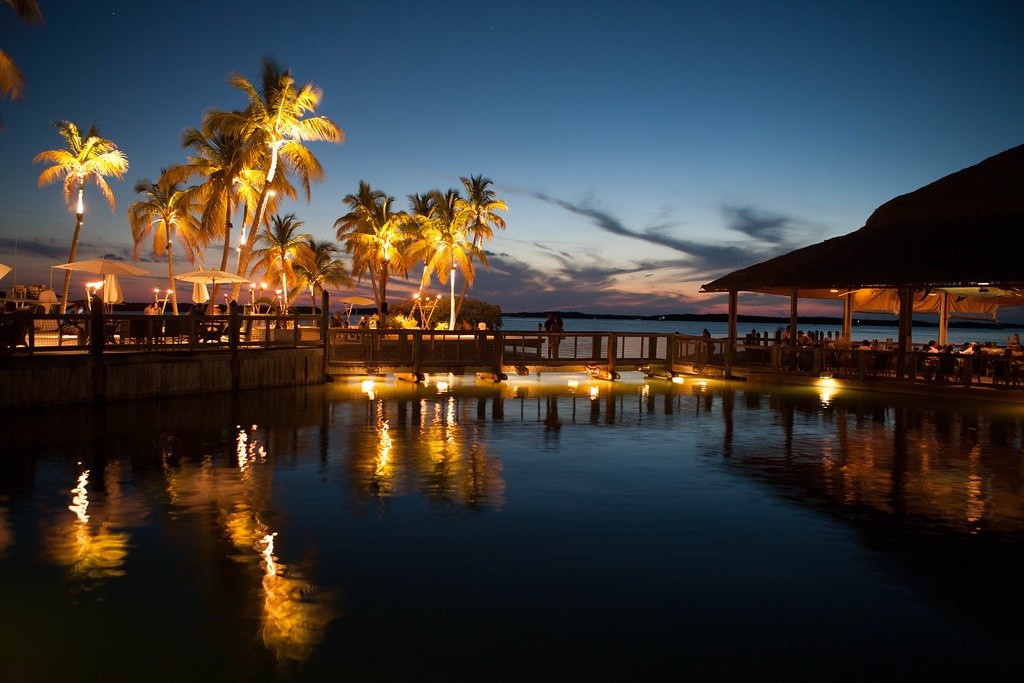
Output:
[337,296,374,323]
[53,258,209,312]
[172,268,249,341]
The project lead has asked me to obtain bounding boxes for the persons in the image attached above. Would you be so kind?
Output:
[60,298,246,346]
[544,311,563,360]
[698,326,1024,389]
[330,311,379,328]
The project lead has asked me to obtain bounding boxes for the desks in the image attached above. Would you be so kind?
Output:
[201,320,228,343]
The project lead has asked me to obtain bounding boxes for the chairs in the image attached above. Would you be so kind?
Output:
[0,309,33,348]
[742,339,1021,386]
[55,319,253,346]
